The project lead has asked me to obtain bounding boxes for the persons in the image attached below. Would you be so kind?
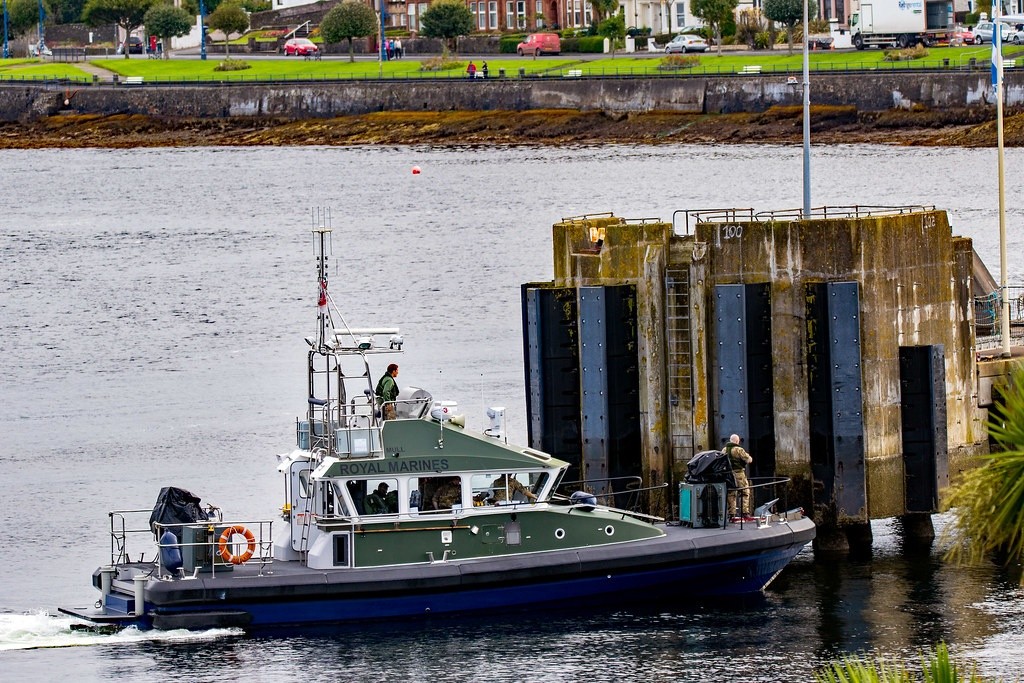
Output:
[432,476,461,514]
[376,364,400,420]
[419,476,438,514]
[466,61,477,82]
[376,36,403,61]
[370,482,394,513]
[720,434,756,522]
[482,60,488,79]
[491,473,538,506]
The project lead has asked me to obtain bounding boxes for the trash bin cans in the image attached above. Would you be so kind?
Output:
[807,35,835,50]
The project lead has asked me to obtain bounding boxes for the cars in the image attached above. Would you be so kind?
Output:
[283,38,319,56]
[1013,28,1024,45]
[665,34,708,53]
[927,24,974,46]
[121,37,142,54]
[972,22,1014,44]
[517,33,562,56]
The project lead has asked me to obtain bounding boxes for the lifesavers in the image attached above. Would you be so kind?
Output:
[219,526,256,565]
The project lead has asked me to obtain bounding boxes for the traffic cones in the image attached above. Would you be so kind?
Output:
[813,39,816,51]
[831,38,835,51]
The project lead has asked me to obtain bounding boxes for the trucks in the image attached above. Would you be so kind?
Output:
[850,0,955,50]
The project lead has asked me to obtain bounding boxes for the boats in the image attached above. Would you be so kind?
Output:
[56,206,818,637]
